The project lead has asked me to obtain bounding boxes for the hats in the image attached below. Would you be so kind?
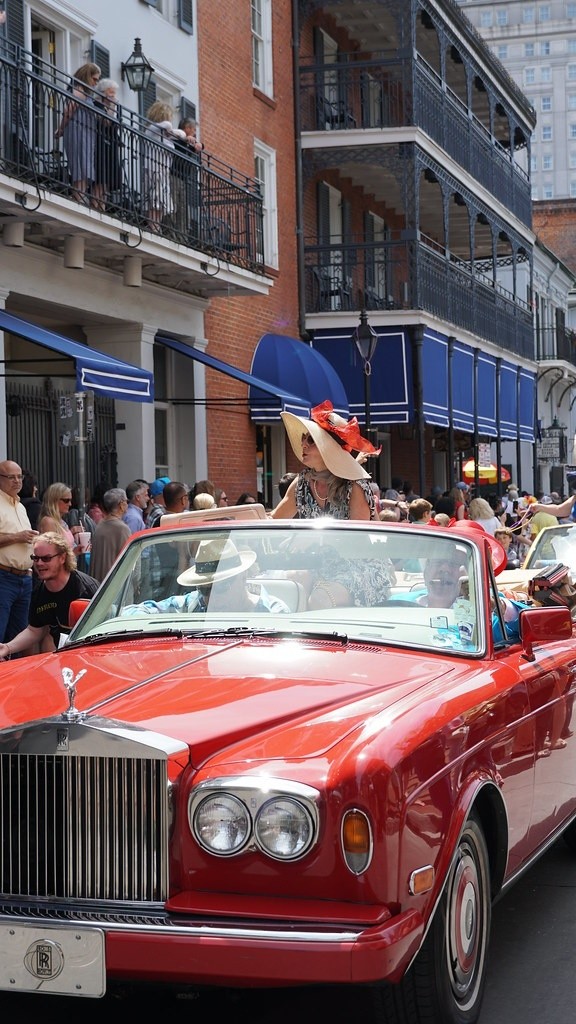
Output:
[506,485,520,492]
[521,491,532,497]
[176,540,257,587]
[508,491,518,501]
[430,486,443,496]
[456,482,470,491]
[280,401,383,480]
[541,496,552,504]
[149,477,171,496]
[550,492,560,500]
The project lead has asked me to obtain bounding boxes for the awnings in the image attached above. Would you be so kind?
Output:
[157,335,311,419]
[0,308,155,404]
[249,334,349,426]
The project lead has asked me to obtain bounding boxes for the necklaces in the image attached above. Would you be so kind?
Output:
[314,480,328,500]
[506,505,536,534]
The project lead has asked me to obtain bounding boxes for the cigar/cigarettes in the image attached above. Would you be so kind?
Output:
[429,580,447,584]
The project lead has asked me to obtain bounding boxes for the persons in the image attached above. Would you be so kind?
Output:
[268,401,397,610]
[417,549,531,652]
[40,483,92,556]
[384,659,576,847]
[55,63,119,211]
[369,481,517,563]
[144,102,204,240]
[0,461,40,658]
[91,477,255,606]
[121,538,290,616]
[279,473,299,519]
[0,531,100,658]
[516,491,568,559]
[530,471,576,523]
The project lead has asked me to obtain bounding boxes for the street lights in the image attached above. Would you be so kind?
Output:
[349,306,378,481]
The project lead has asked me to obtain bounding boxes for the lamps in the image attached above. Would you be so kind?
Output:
[121,37,155,93]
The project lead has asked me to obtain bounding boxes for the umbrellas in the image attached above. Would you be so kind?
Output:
[462,458,511,484]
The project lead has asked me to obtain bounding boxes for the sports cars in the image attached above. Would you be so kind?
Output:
[0,516,576,1024]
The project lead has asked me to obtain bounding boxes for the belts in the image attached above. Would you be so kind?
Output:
[0,564,30,576]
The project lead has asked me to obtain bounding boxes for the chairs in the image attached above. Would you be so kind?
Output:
[248,578,307,614]
[10,130,71,196]
[320,95,357,130]
[109,182,254,265]
[364,288,399,311]
[309,264,352,313]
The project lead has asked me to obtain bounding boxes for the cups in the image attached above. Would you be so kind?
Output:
[79,532,92,554]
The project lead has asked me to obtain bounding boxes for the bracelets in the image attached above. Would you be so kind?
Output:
[395,501,399,507]
[5,643,11,658]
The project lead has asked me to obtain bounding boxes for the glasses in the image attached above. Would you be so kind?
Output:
[301,431,318,445]
[196,577,236,596]
[30,552,63,562]
[220,497,229,501]
[0,475,25,481]
[59,498,72,503]
